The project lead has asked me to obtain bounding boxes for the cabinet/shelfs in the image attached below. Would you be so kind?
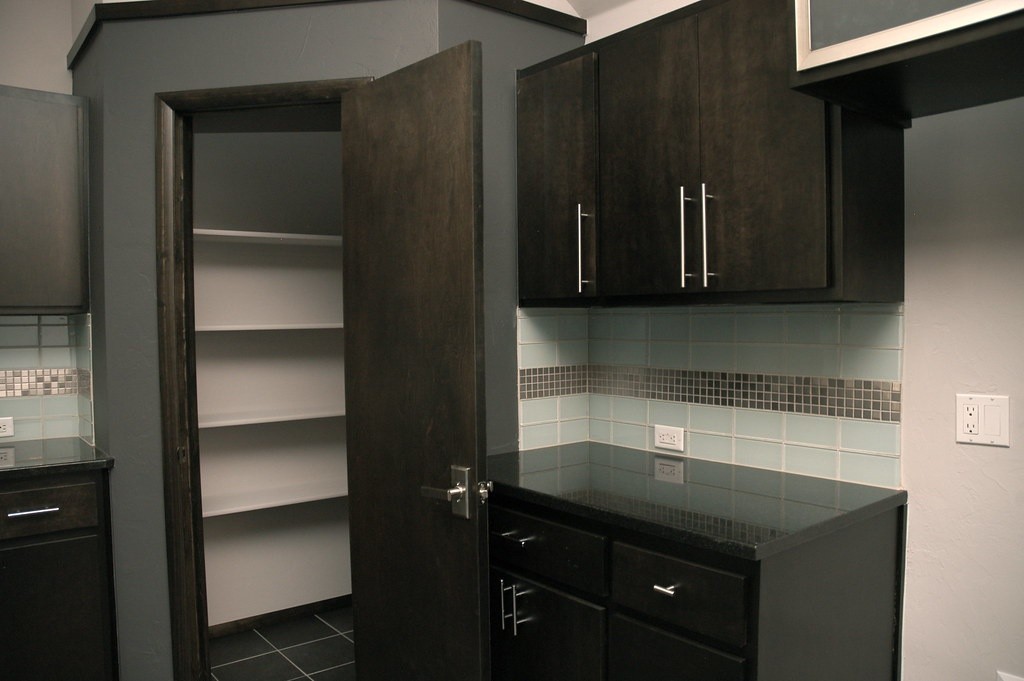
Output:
[598,0,908,309]
[0,472,119,681]
[0,84,89,315]
[513,42,597,308]
[484,504,907,681]
[193,227,351,630]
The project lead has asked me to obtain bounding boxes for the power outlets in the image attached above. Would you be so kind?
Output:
[0,417,14,437]
[654,424,685,451]
[956,393,1010,448]
[651,457,684,485]
[0,447,15,469]
